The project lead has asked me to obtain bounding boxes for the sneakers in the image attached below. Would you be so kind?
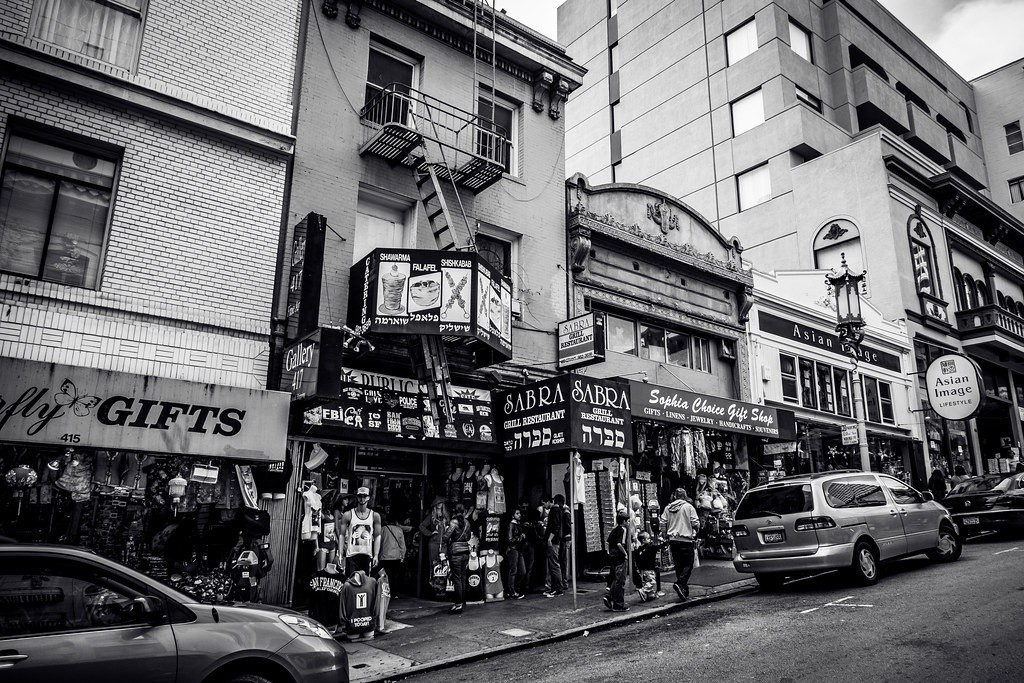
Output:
[638,590,647,602]
[504,593,513,600]
[547,591,563,598]
[448,605,460,613]
[613,604,630,611]
[452,606,463,614]
[604,596,612,610]
[672,583,686,602]
[649,592,660,600]
[685,587,689,598]
[513,592,525,599]
[542,589,553,596]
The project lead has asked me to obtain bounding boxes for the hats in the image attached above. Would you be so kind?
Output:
[431,495,449,508]
[319,488,337,501]
[357,487,371,496]
[336,493,354,502]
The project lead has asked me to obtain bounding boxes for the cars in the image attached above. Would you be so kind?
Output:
[943,470,1024,536]
[0,540,351,682]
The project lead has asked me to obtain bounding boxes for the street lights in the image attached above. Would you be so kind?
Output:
[825,251,874,482]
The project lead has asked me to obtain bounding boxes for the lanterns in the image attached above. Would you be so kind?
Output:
[6,461,38,516]
[168,472,187,516]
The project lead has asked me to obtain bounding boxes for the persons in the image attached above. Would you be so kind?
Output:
[534,493,572,600]
[441,504,471,616]
[227,529,272,603]
[506,508,528,601]
[927,469,947,502]
[299,475,392,642]
[950,465,970,493]
[633,530,670,602]
[601,513,631,613]
[659,487,702,601]
[1005,450,1018,473]
[1015,456,1024,474]
[378,513,406,602]
[396,461,506,606]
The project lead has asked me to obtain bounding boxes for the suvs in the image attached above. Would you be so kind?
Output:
[730,467,964,591]
